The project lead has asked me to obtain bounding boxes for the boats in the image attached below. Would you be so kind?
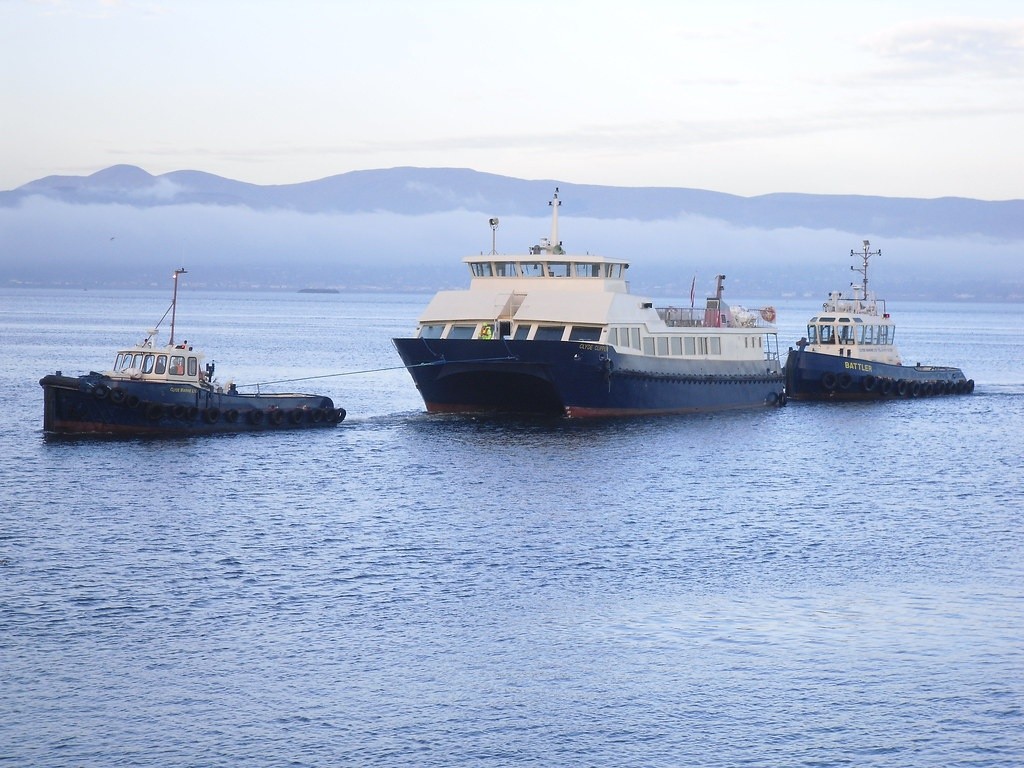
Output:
[39,264,346,442]
[781,239,975,401]
[391,186,787,423]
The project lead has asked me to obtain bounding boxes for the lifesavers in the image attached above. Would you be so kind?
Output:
[761,307,775,321]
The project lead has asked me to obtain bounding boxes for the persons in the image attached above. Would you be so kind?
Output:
[173,359,183,374]
[479,323,492,339]
[830,330,840,342]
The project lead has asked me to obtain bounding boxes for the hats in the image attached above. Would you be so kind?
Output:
[483,322,487,326]
